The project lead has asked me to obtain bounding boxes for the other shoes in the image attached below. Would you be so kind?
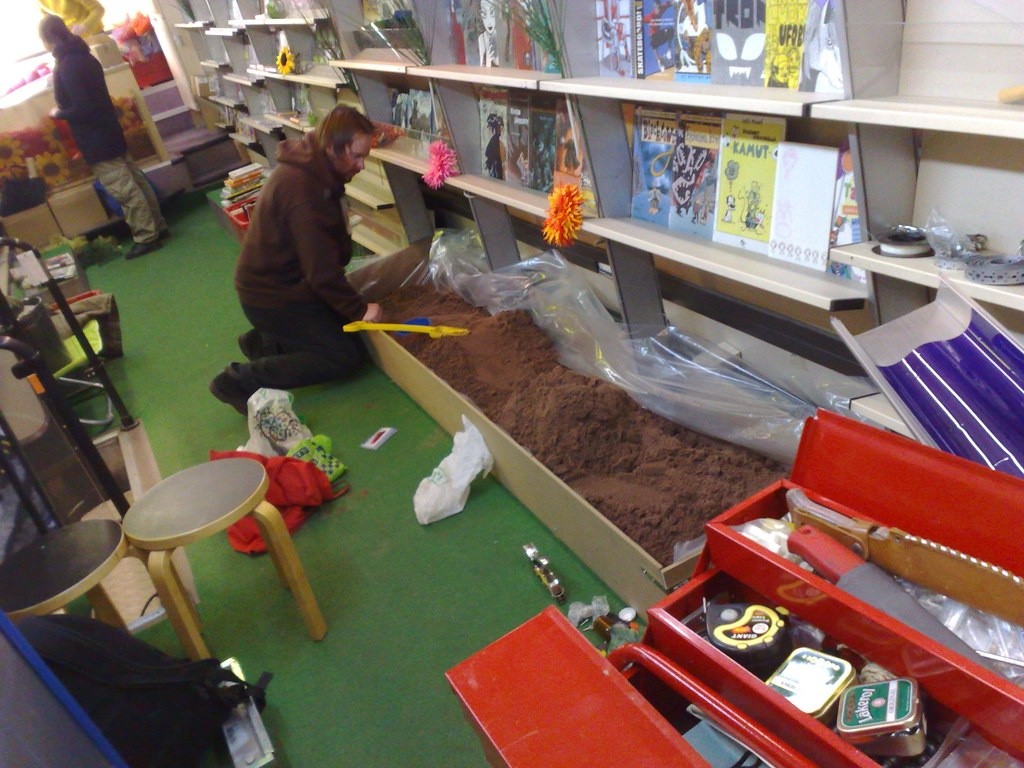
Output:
[209,362,249,417]
[237,329,273,363]
[158,229,170,241]
[125,239,163,260]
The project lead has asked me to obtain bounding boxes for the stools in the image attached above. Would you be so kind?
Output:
[122,458,326,664]
[0,517,205,640]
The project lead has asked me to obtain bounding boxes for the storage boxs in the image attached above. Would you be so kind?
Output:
[30,244,91,304]
[343,237,1024,768]
[206,189,257,242]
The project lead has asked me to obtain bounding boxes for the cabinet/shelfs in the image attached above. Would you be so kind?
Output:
[174,0,1024,444]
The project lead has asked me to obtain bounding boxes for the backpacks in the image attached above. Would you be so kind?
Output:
[18,615,271,768]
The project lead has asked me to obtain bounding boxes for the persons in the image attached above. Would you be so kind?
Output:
[38,14,173,261]
[210,101,384,415]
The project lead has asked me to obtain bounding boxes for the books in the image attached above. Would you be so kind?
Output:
[478,85,596,206]
[219,162,268,222]
[216,103,255,141]
[387,87,434,143]
[619,99,868,285]
[594,0,845,94]
[449,0,562,74]
[362,0,421,30]
[290,116,311,127]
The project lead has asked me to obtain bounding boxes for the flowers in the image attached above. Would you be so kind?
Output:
[0,94,135,186]
[274,47,297,74]
[420,139,461,189]
[540,183,586,247]
